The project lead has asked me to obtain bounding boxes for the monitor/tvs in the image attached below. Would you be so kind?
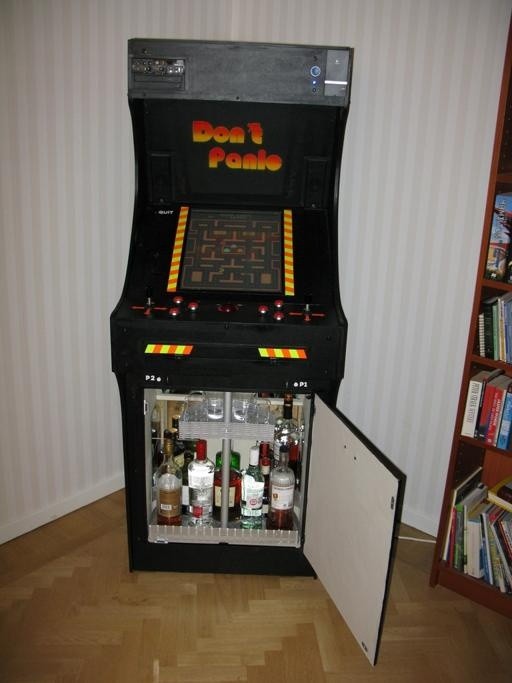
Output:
[176,206,289,302]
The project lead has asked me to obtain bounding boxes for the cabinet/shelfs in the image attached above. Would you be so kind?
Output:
[426,14,511,617]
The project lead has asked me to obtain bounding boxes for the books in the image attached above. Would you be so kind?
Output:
[441,190,512,595]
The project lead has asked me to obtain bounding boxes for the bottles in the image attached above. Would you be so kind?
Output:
[151,393,305,537]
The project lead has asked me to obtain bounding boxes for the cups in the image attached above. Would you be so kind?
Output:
[181,391,272,425]
